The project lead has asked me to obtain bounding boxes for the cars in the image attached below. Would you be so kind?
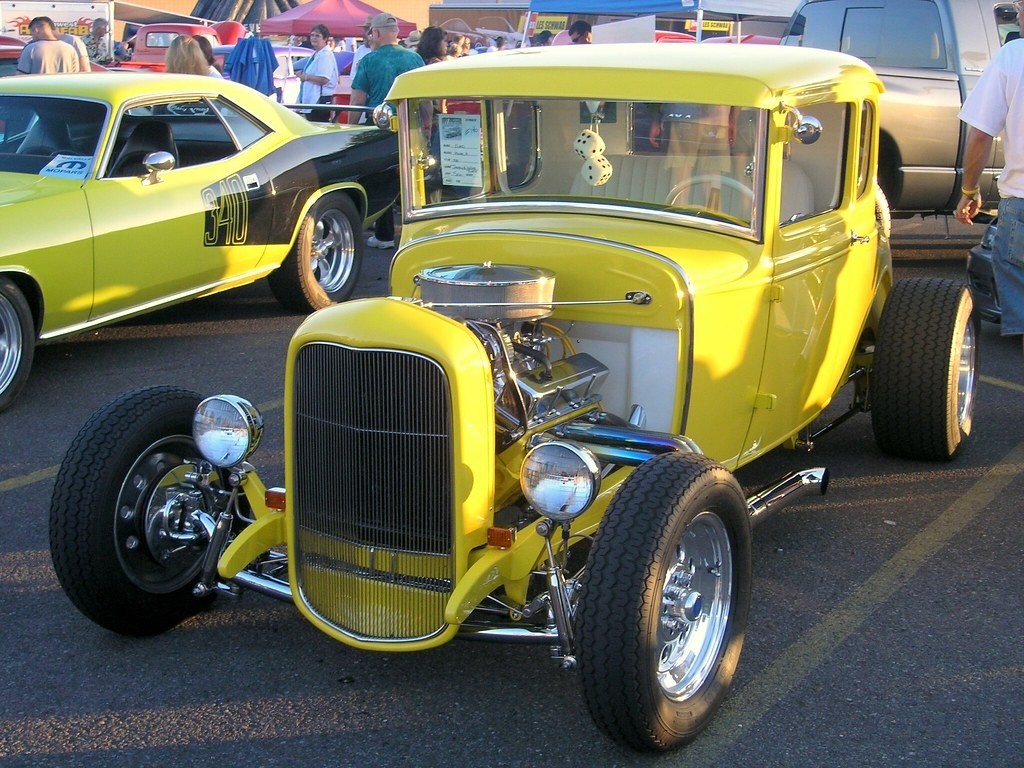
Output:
[46,42,982,755]
[0,68,435,415]
[0,20,788,127]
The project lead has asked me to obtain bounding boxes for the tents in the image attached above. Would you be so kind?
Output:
[520,0,800,44]
[259,0,417,48]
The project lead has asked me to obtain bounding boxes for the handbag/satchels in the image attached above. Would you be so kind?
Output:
[309,86,334,122]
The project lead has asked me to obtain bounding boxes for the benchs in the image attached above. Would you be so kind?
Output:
[567,152,816,228]
[112,138,234,170]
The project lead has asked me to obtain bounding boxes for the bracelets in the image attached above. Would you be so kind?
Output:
[962,185,980,194]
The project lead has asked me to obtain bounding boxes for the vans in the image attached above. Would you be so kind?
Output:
[780,0,1024,227]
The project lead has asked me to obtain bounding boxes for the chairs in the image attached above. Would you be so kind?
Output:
[17,99,76,159]
[109,120,181,182]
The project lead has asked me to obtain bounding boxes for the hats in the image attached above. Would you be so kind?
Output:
[367,12,398,35]
[538,31,552,46]
[356,16,373,27]
[405,31,421,45]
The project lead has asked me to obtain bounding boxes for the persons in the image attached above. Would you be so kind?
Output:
[291,24,357,124]
[16,16,91,73]
[954,0,1024,341]
[539,30,554,46]
[569,20,592,44]
[164,33,224,79]
[80,18,112,62]
[348,11,433,249]
[649,101,740,213]
[416,24,524,201]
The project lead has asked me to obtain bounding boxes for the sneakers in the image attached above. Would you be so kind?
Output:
[367,235,395,249]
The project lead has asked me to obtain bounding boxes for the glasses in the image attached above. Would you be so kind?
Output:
[572,33,585,42]
